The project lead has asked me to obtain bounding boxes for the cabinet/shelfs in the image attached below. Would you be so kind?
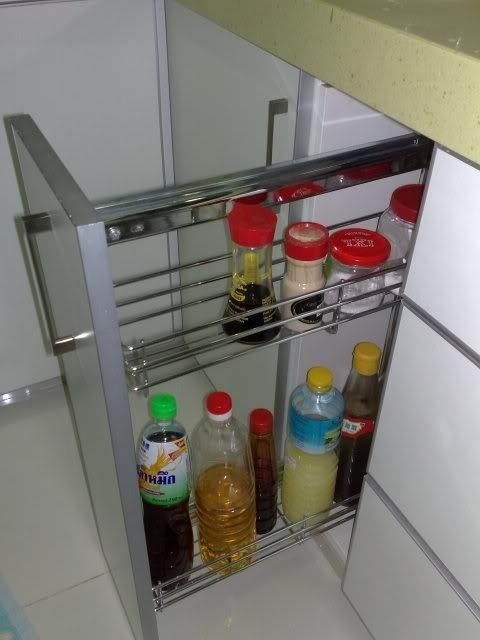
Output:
[2,2,480,640]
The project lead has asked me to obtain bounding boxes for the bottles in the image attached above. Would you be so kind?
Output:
[137,393,195,592]
[191,391,257,577]
[221,202,282,344]
[282,365,346,528]
[281,221,330,333]
[377,185,426,296]
[248,407,278,534]
[334,338,381,508]
[324,228,391,317]
